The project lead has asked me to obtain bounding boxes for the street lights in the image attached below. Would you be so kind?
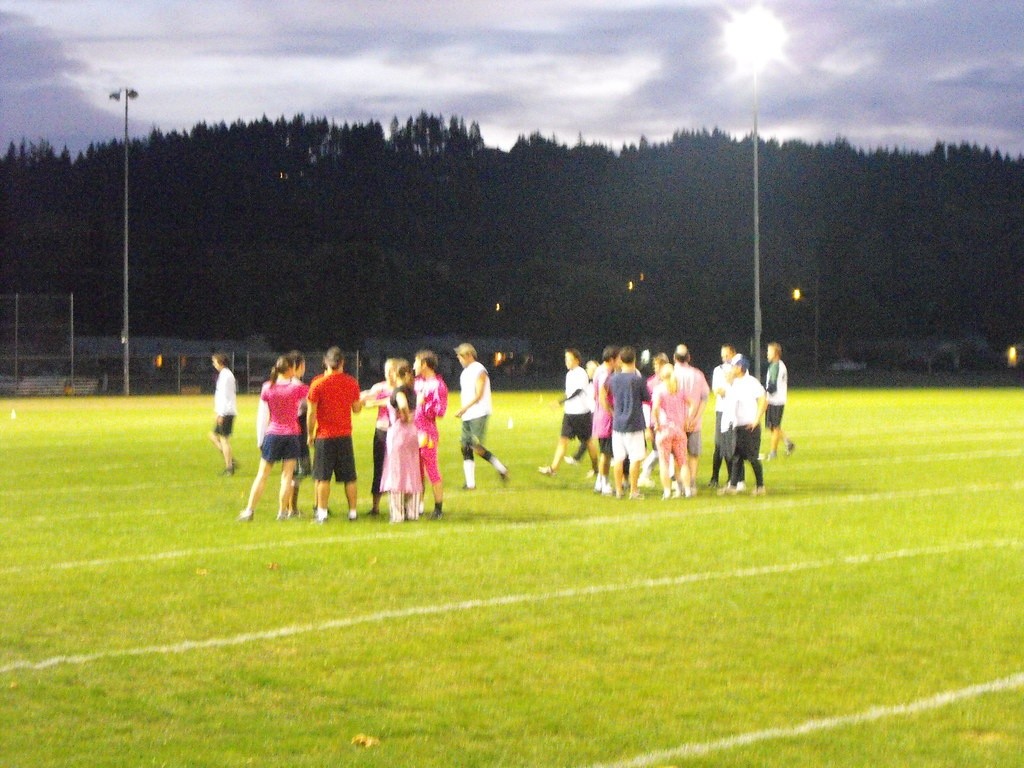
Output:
[107,87,139,396]
[720,7,787,384]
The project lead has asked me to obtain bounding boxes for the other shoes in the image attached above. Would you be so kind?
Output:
[786,443,794,455]
[368,508,379,515]
[593,487,602,492]
[672,481,678,489]
[736,481,745,490]
[602,489,625,496]
[432,511,444,520]
[239,511,253,520]
[539,466,556,475]
[637,477,656,488]
[564,456,574,464]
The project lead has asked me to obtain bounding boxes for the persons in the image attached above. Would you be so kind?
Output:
[707,342,767,498]
[455,343,512,492]
[539,344,710,501]
[210,352,241,476]
[765,341,796,462]
[238,346,448,523]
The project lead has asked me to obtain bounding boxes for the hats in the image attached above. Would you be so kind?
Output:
[454,343,478,359]
[727,354,750,372]
